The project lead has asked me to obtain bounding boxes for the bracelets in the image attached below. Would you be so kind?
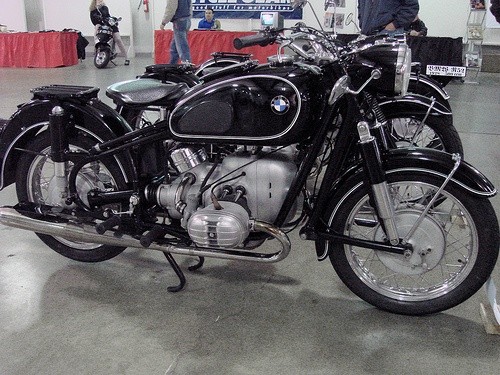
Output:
[162,22,166,26]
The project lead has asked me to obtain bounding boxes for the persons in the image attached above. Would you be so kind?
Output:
[404,15,428,37]
[358,0,419,37]
[198,8,215,29]
[160,0,193,64]
[90,0,130,65]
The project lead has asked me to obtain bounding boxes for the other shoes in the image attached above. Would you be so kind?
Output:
[124,58,130,66]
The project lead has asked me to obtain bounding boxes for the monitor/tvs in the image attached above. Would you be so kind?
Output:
[260,12,284,28]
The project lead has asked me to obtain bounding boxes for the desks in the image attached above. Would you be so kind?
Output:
[335,34,465,83]
[154,30,284,65]
[0,31,79,68]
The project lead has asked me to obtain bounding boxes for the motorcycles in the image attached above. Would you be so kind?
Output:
[0,0,500,315]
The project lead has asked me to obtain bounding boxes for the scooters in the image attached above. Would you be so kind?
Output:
[93,5,122,69]
